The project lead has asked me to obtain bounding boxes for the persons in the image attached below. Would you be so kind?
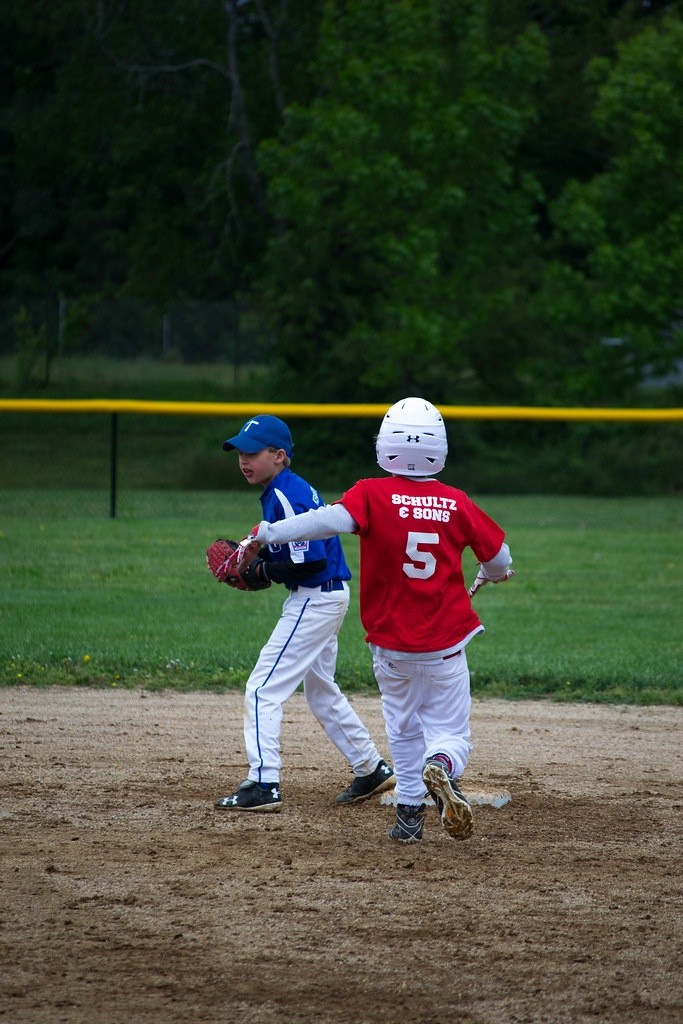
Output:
[211,415,397,813]
[223,395,517,847]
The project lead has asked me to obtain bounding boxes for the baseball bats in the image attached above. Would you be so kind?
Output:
[466,569,486,598]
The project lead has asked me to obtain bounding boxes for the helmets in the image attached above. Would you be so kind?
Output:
[376,397,448,476]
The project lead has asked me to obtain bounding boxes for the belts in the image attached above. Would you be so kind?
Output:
[291,580,344,592]
[442,649,461,660]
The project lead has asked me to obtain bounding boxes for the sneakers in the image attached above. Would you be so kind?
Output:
[334,759,396,805]
[214,778,283,812]
[422,757,473,841]
[388,803,427,844]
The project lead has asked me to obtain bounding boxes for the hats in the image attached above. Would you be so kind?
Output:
[222,414,294,459]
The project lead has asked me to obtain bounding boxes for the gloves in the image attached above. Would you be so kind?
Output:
[215,524,262,583]
[468,562,515,598]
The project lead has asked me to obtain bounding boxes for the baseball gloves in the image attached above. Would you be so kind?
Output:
[205,538,272,592]
